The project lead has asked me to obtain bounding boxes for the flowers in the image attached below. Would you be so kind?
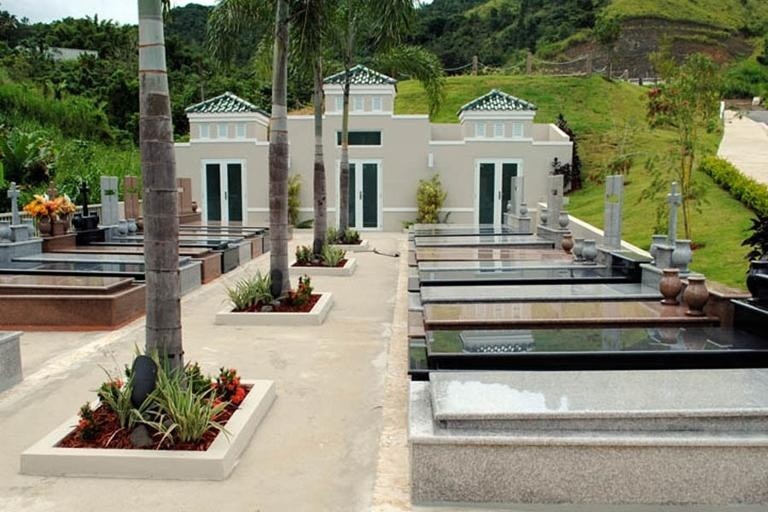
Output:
[75,367,247,449]
[21,193,76,219]
[290,276,314,311]
